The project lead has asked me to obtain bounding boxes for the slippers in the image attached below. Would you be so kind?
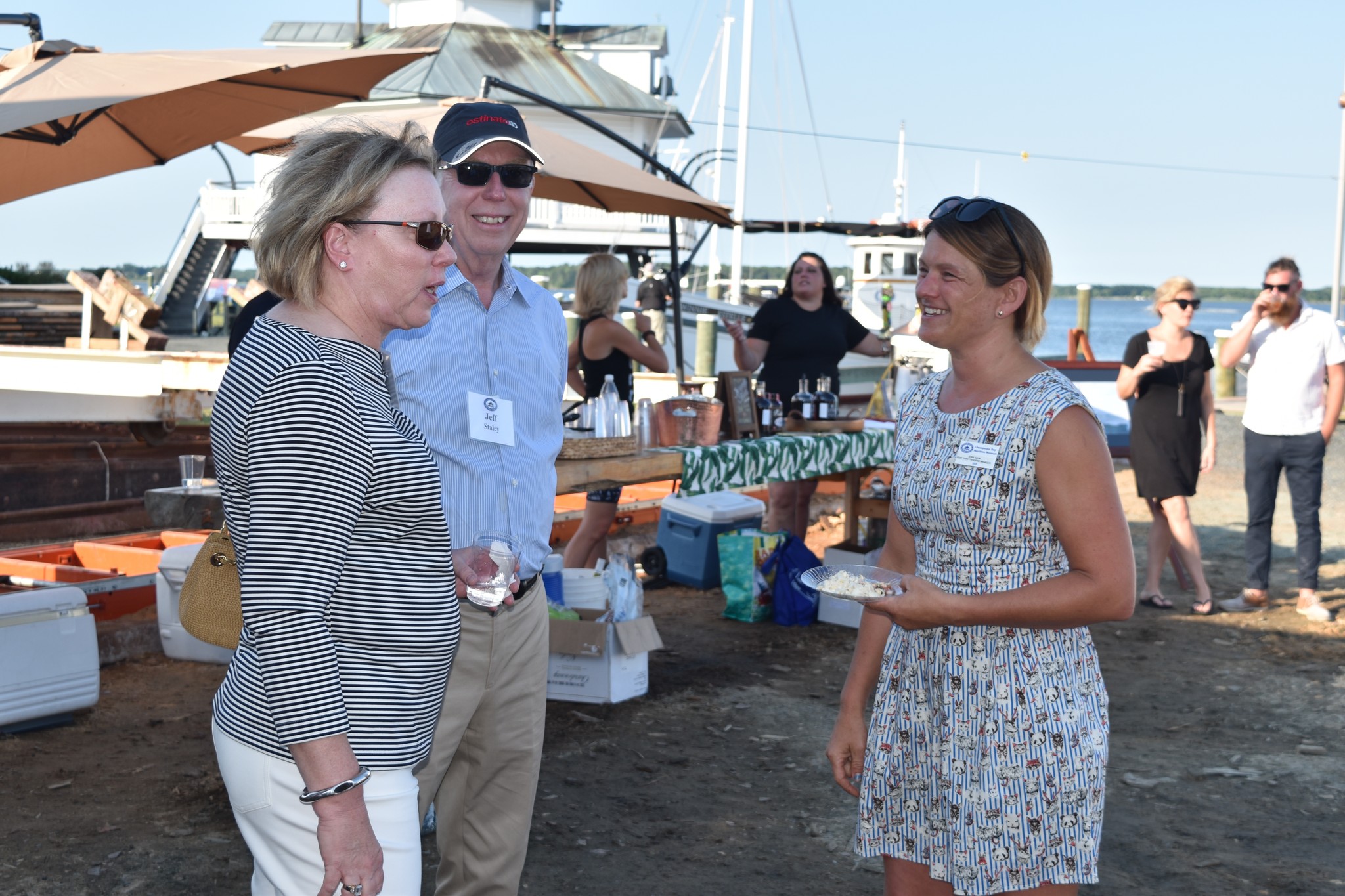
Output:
[1191,595,1215,614]
[1139,594,1172,609]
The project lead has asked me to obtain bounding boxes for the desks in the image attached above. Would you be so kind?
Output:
[146,418,895,543]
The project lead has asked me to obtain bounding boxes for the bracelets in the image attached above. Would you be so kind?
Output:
[880,340,893,354]
[300,765,372,805]
[643,329,656,340]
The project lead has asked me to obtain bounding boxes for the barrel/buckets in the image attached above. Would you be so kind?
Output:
[541,563,563,607]
[655,393,725,447]
[561,567,610,610]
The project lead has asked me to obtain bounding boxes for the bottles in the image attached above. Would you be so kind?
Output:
[753,380,784,436]
[596,373,621,439]
[791,377,840,421]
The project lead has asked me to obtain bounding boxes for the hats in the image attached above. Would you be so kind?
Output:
[432,102,544,171]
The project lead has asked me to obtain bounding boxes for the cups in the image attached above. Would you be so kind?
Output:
[1266,291,1287,313]
[178,454,206,490]
[1148,339,1165,361]
[634,399,658,457]
[575,396,632,437]
[465,530,524,606]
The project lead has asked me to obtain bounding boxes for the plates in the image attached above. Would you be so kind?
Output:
[800,564,907,602]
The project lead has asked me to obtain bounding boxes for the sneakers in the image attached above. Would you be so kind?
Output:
[1219,585,1268,613]
[1295,592,1332,621]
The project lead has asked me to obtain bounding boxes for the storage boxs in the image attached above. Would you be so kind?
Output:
[654,490,769,590]
[0,587,101,736]
[155,546,237,664]
[818,537,889,629]
[546,608,666,705]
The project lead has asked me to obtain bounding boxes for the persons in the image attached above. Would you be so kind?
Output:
[208,122,518,896]
[719,252,896,551]
[562,254,668,570]
[825,194,1137,896]
[376,101,566,895]
[1117,277,1218,614]
[1215,257,1345,621]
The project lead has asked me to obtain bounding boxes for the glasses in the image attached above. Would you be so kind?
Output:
[928,196,1026,277]
[438,158,539,188]
[1165,298,1201,310]
[337,216,456,250]
[1265,279,1296,292]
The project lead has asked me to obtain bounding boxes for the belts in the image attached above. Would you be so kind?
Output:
[511,563,546,600]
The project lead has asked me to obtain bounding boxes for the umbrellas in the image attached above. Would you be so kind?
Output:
[0,32,438,207]
[220,95,739,237]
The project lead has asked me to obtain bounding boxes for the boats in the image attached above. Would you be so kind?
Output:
[630,0,951,427]
[0,12,446,546]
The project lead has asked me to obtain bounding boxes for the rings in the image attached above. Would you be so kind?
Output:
[343,884,365,896]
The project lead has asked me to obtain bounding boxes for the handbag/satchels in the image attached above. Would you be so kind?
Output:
[716,521,791,622]
[763,534,826,627]
